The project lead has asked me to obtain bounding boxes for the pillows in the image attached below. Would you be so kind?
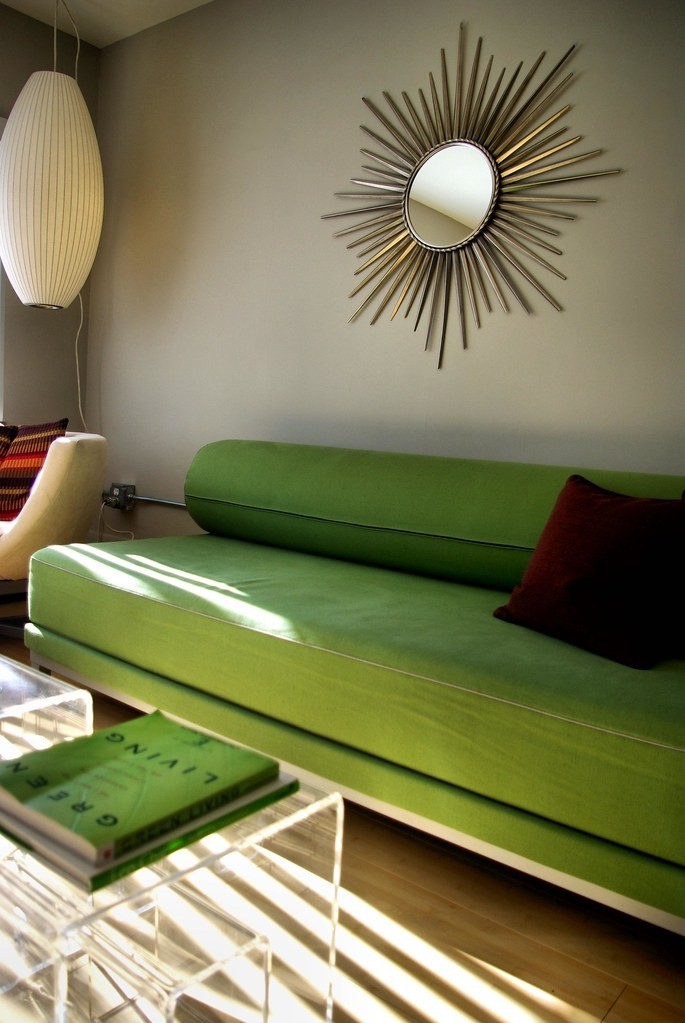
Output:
[494,476,684,670]
[0,418,69,520]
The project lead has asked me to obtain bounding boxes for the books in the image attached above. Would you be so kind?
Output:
[0,705,299,896]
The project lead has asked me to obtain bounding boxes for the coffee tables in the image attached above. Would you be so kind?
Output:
[0,653,344,1023]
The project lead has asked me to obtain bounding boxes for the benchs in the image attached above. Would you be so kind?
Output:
[0,432,109,581]
[23,439,684,939]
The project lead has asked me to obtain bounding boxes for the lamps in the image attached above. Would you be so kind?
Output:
[0,0,104,310]
